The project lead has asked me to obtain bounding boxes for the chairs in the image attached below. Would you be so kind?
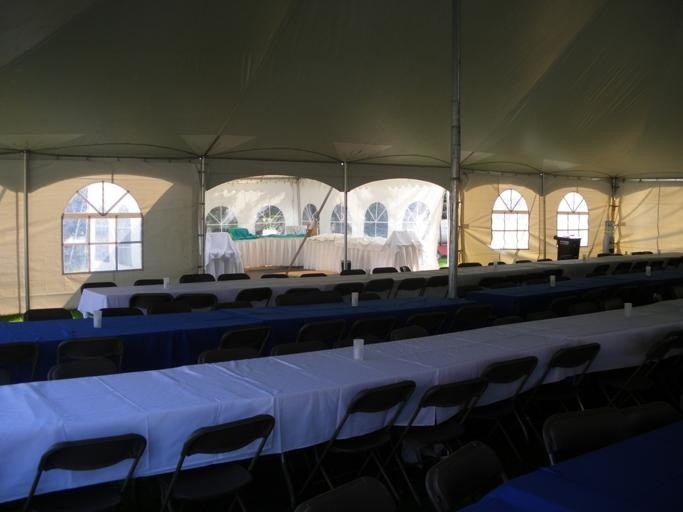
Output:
[652,278,682,302]
[648,261,664,271]
[129,293,174,307]
[542,406,631,463]
[217,273,251,281]
[234,287,272,307]
[303,377,417,505]
[390,324,427,339]
[450,304,493,331]
[334,282,363,294]
[600,330,682,408]
[179,273,215,284]
[176,292,218,312]
[340,268,365,275]
[276,294,305,304]
[133,278,164,285]
[512,342,601,460]
[425,439,508,512]
[296,478,400,512]
[600,284,650,308]
[479,275,517,289]
[523,275,567,285]
[309,292,344,303]
[405,311,447,335]
[160,414,276,512]
[632,261,648,274]
[22,433,147,512]
[80,282,116,294]
[451,355,552,481]
[665,256,681,269]
[196,325,294,364]
[586,264,609,277]
[22,308,73,320]
[394,277,426,300]
[211,301,251,308]
[51,337,124,380]
[100,307,143,317]
[399,265,411,272]
[284,287,320,294]
[295,319,346,351]
[548,296,601,315]
[424,275,450,297]
[373,267,397,273]
[365,278,394,301]
[147,301,192,314]
[384,378,489,502]
[613,261,632,273]
[348,315,398,345]
[357,293,380,302]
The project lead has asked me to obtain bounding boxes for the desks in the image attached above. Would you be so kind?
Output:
[83,252,682,316]
[1,271,681,371]
[0,299,682,502]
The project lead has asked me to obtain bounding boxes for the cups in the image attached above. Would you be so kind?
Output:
[352,293,358,307]
[624,303,632,320]
[93,311,102,328]
[550,276,556,287]
[164,278,170,288]
[645,266,651,276]
[353,339,364,360]
